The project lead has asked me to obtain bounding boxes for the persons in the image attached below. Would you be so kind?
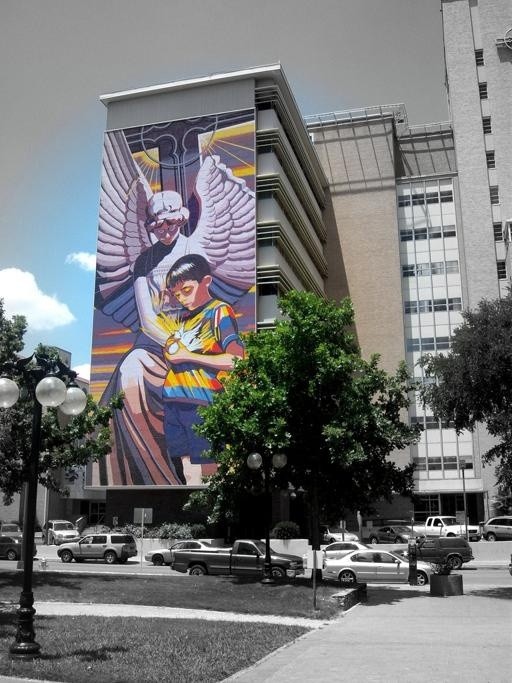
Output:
[160,253,246,485]
[97,188,218,485]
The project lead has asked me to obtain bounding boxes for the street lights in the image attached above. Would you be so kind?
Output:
[248,448,286,584]
[1,373,90,660]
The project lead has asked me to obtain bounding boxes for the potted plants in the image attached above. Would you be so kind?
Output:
[430,562,462,597]
